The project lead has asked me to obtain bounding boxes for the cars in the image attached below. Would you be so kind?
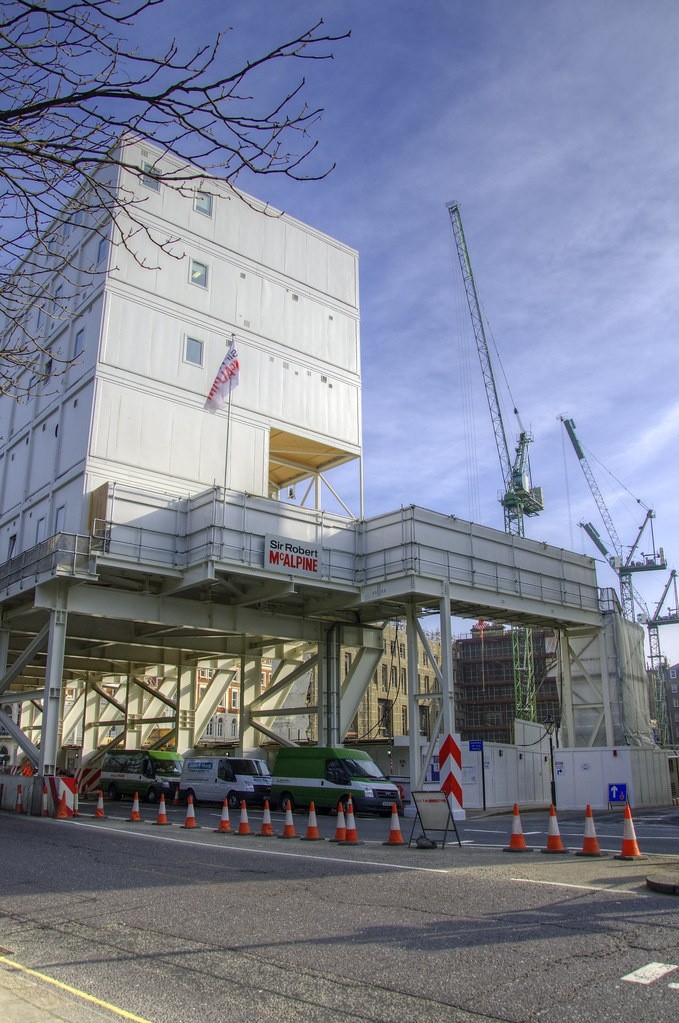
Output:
[274,747,403,815]
[99,750,188,807]
[178,756,271,807]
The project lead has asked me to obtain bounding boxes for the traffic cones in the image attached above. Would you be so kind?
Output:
[125,792,144,822]
[277,799,302,838]
[256,800,274,837]
[330,794,365,842]
[540,804,571,854]
[383,803,409,847]
[181,796,200,831]
[172,786,180,807]
[91,790,107,821]
[502,804,535,855]
[574,804,608,857]
[150,794,173,826]
[232,800,254,836]
[212,798,233,834]
[299,802,323,840]
[614,804,648,860]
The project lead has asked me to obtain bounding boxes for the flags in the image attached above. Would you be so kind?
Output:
[204,339,239,414]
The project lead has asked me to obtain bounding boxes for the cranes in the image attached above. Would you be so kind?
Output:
[642,569,678,763]
[446,198,541,538]
[560,414,665,624]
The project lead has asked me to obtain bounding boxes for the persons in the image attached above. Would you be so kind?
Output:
[328,762,344,778]
